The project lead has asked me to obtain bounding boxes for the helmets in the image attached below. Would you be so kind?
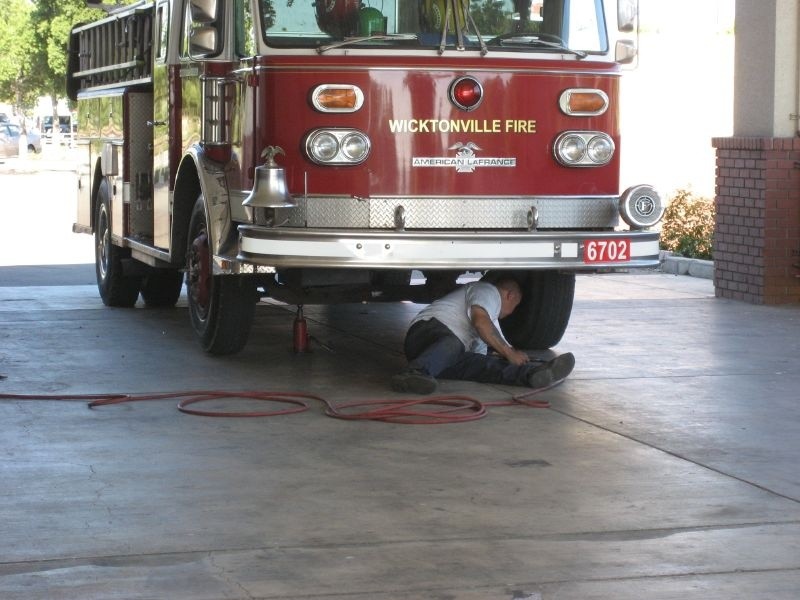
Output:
[351,8,387,37]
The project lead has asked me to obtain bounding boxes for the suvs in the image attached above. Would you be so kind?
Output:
[39,115,72,145]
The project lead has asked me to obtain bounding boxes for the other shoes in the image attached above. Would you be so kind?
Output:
[523,352,575,388]
[391,368,437,394]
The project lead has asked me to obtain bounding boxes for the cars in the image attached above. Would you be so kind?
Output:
[0,121,43,159]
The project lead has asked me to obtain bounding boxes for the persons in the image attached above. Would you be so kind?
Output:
[390,270,575,395]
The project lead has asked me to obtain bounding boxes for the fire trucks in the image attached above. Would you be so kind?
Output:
[66,0,661,358]
